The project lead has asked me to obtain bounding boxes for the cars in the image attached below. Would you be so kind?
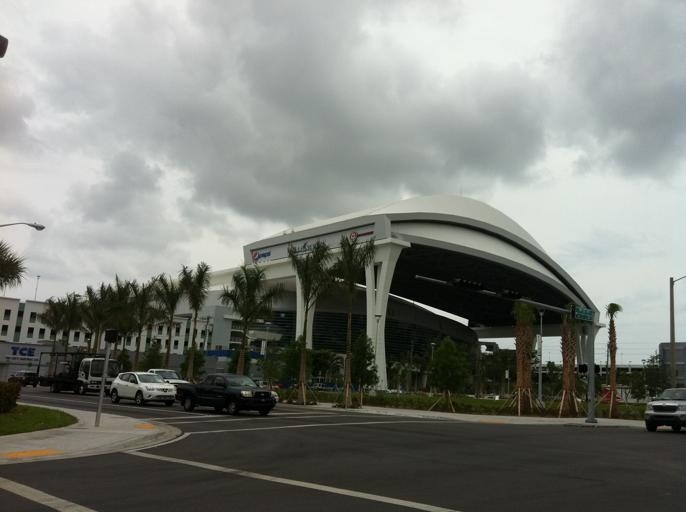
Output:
[596,387,623,402]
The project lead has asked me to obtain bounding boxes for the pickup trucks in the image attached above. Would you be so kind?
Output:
[174,373,280,416]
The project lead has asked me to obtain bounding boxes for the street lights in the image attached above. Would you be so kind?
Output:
[474,345,488,399]
[0,222,45,231]
[373,314,382,366]
[428,343,437,361]
[262,321,272,360]
[536,308,546,400]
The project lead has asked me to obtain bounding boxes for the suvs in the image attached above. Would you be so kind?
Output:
[109,371,174,407]
[148,369,190,396]
[645,387,686,433]
[8,370,36,387]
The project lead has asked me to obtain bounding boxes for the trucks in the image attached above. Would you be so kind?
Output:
[33,352,123,396]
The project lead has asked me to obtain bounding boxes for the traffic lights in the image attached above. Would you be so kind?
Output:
[496,287,523,300]
[449,277,485,292]
